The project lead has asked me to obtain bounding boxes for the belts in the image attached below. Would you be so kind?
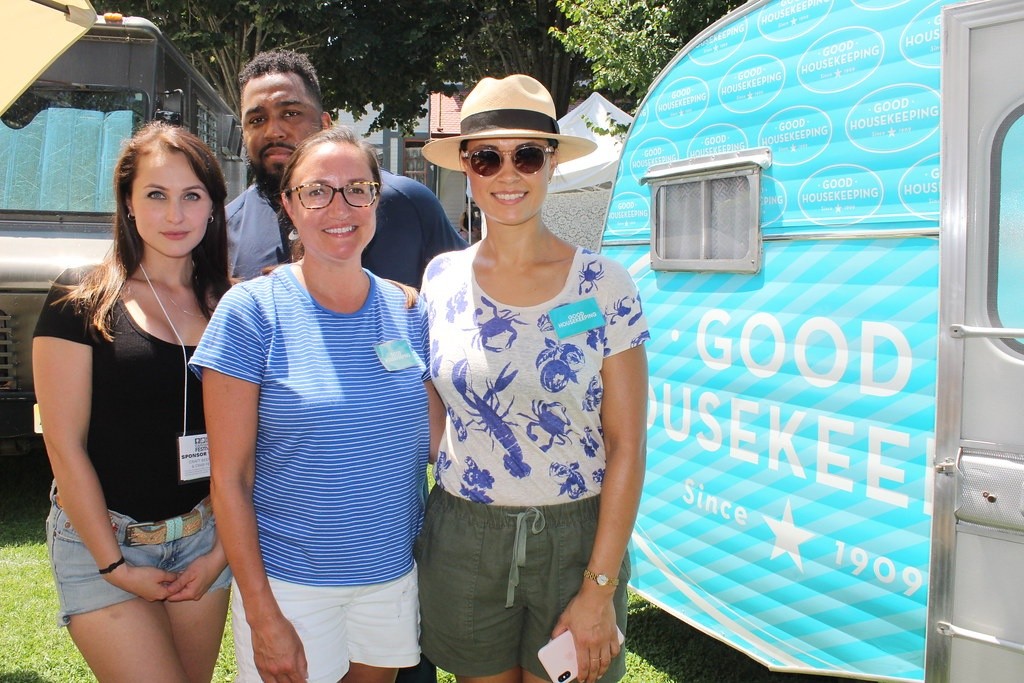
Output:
[54,491,213,547]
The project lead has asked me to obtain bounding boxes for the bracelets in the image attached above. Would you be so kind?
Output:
[99,555,125,575]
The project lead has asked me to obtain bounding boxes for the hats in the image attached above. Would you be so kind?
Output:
[421,75,598,171]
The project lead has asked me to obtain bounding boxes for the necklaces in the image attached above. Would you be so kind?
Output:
[167,295,205,317]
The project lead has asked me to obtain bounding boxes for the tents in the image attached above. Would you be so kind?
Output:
[467,92,634,245]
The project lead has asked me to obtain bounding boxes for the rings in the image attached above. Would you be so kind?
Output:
[592,658,601,661]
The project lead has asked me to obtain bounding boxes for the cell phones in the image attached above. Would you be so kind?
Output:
[538,625,625,683]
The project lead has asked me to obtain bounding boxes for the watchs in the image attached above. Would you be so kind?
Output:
[584,569,620,588]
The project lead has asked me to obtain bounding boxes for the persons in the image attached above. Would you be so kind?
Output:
[30,119,240,683]
[460,203,477,232]
[226,46,473,683]
[186,124,447,683]
[417,73,647,683]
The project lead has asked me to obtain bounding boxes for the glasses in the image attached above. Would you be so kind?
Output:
[285,176,381,209]
[460,144,558,178]
